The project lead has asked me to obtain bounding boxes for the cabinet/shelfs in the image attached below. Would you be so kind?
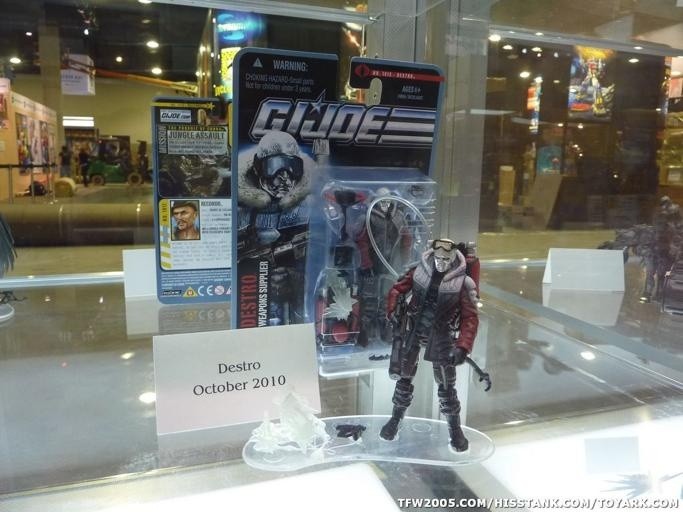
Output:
[480,39,667,231]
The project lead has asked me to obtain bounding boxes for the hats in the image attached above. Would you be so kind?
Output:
[172,200,198,213]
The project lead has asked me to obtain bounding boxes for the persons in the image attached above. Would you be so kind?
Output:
[78,144,92,187]
[379,237,478,452]
[170,199,201,241]
[136,151,150,181]
[56,144,75,177]
[238,128,319,323]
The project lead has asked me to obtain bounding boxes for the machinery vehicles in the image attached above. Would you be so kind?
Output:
[86,137,153,185]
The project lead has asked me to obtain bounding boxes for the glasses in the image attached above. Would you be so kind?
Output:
[253,154,305,182]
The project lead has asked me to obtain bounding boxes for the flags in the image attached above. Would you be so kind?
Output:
[321,172,431,355]
